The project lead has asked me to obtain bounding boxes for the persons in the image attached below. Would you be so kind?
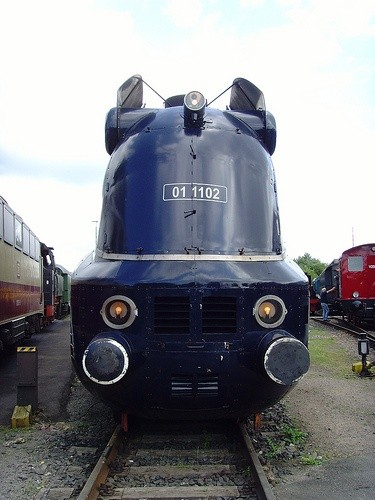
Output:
[320,286,336,322]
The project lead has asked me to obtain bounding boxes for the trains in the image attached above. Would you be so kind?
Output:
[309,243,375,326]
[70,76,309,420]
[0,196,72,354]
